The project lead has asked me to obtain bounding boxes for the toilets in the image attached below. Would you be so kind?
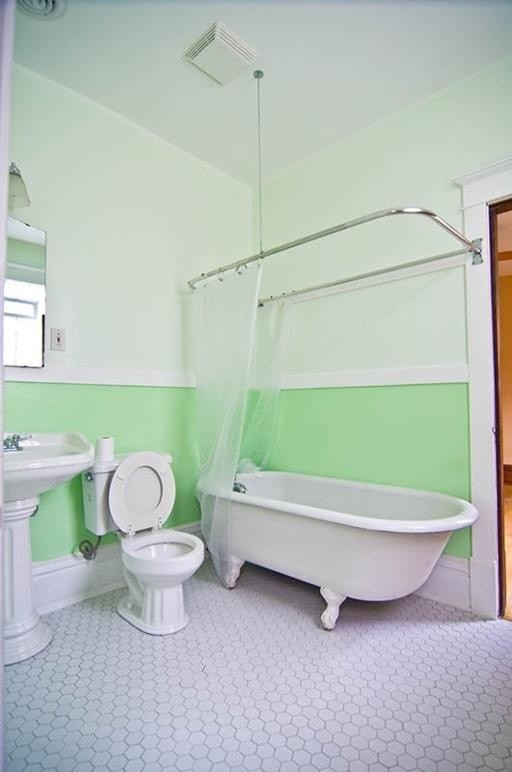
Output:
[80,449,206,637]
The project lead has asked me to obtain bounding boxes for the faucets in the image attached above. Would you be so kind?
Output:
[3,438,13,450]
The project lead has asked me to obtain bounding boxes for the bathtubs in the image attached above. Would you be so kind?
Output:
[196,470,480,632]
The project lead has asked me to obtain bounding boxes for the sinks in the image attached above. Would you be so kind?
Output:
[0,431,95,504]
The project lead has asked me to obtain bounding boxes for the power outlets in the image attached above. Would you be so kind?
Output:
[51,327,67,352]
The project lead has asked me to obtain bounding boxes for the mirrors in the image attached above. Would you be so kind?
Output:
[5,217,47,369]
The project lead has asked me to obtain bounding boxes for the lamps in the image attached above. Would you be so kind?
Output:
[7,162,32,219]
